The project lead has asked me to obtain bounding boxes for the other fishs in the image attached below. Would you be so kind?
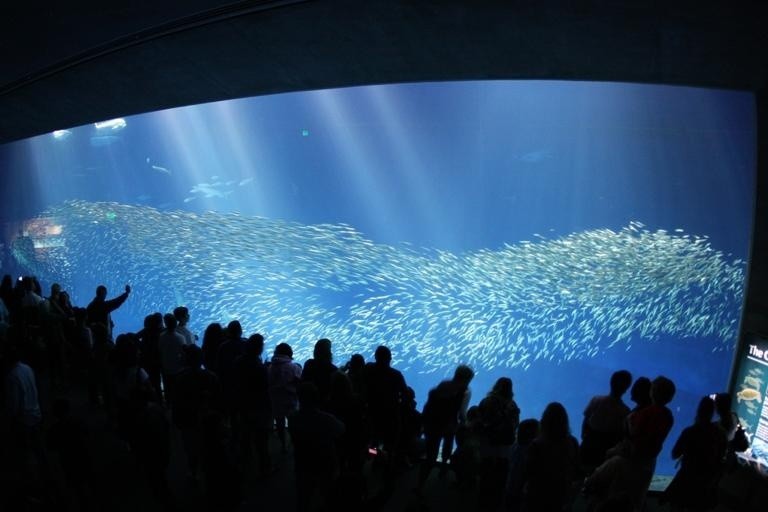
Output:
[9,196,750,377]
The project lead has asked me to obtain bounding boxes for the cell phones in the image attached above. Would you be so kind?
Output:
[368,447,378,454]
